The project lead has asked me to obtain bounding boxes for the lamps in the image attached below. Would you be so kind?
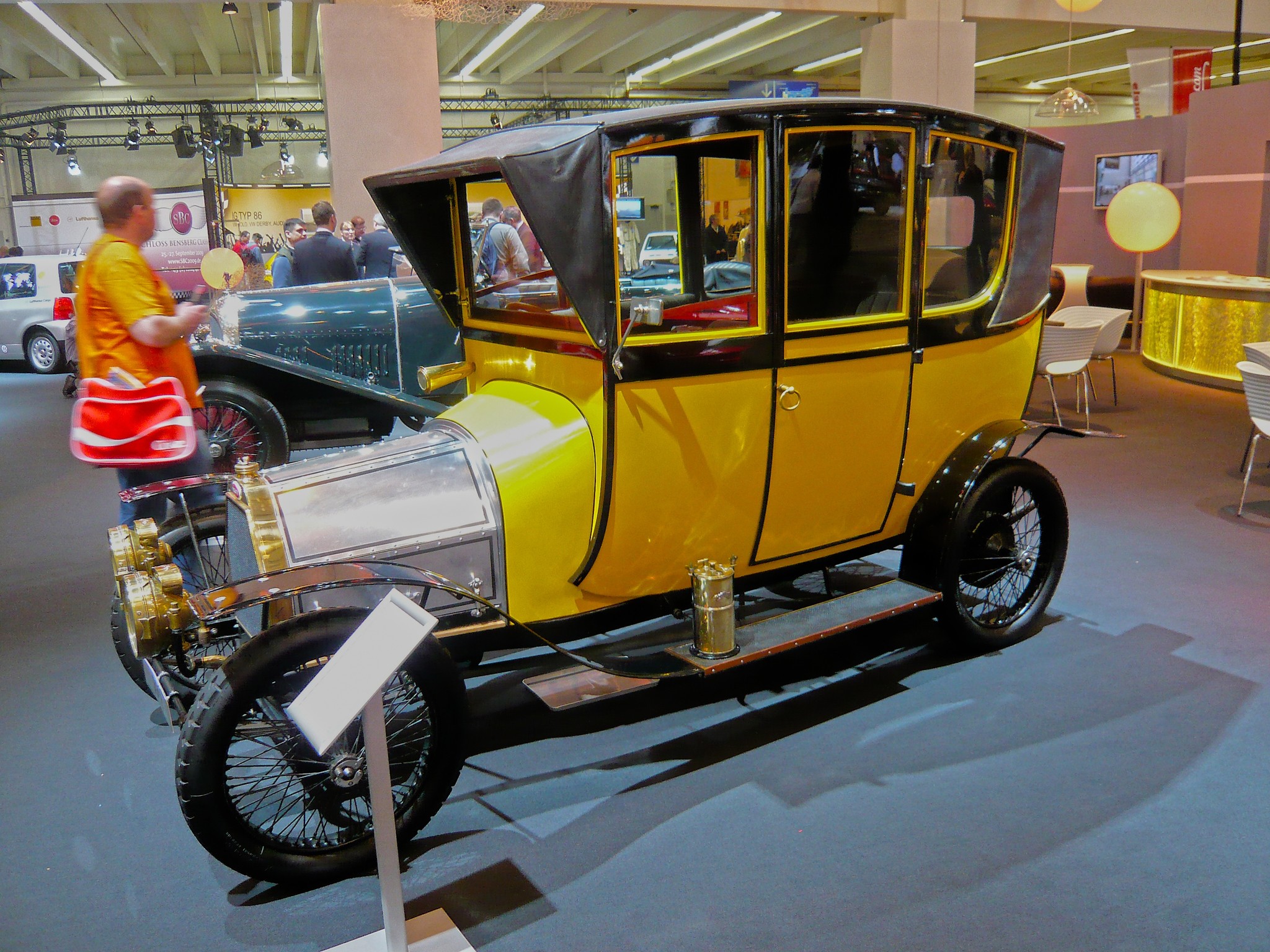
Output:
[222,2,238,16]
[1033,1,1101,120]
[20,111,330,175]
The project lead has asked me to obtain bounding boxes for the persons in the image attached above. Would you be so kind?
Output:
[355,212,403,281]
[470,198,531,309]
[241,232,265,291]
[705,213,729,264]
[271,217,308,288]
[619,220,641,275]
[727,233,740,260]
[62,292,82,399]
[232,230,251,291]
[350,215,368,274]
[338,220,365,280]
[789,141,1001,296]
[291,200,360,286]
[0,244,24,266]
[73,175,225,597]
[500,205,541,284]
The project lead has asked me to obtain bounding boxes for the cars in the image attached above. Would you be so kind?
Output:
[108,96,1105,888]
[0,253,88,374]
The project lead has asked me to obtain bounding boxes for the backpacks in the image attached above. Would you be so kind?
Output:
[469,221,512,285]
[242,244,259,266]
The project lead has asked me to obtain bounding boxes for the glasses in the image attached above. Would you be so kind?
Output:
[355,226,367,231]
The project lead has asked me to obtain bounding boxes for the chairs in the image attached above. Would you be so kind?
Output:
[1234,360,1270,516]
[1037,321,1105,434]
[1239,340,1270,474]
[1045,304,1133,412]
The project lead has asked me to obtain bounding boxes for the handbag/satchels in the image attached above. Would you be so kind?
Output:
[68,368,197,466]
